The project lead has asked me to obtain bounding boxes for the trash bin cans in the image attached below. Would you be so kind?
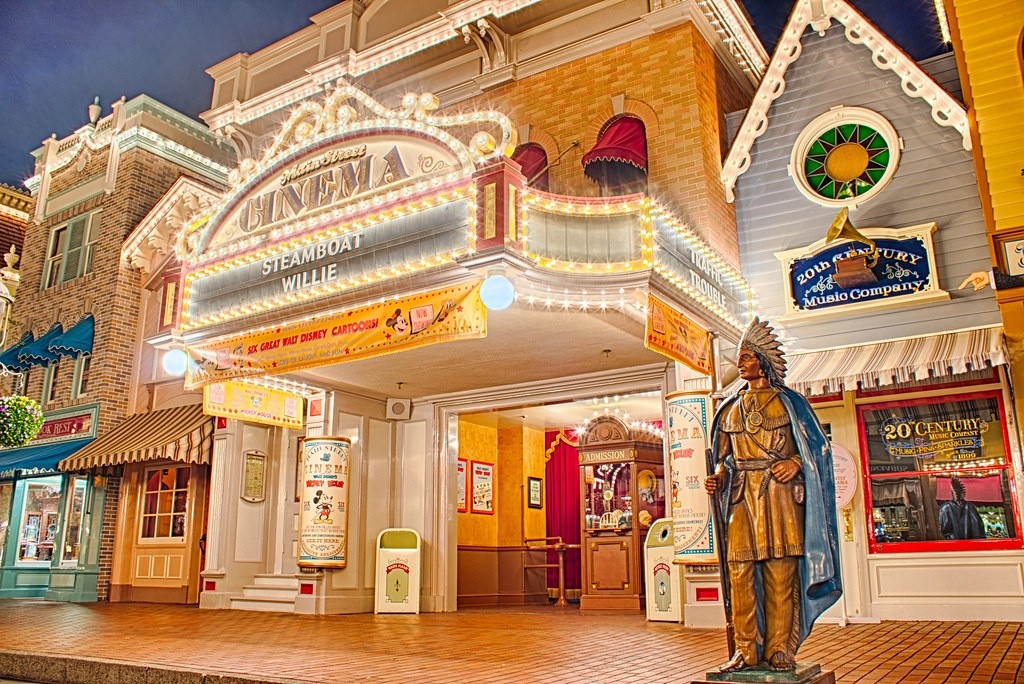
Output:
[374,528,422,615]
[644,517,687,624]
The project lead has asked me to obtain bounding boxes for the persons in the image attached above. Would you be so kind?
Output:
[938,477,988,540]
[704,315,850,671]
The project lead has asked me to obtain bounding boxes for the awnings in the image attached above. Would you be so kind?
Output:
[511,146,548,192]
[47,314,94,358]
[581,118,648,188]
[17,324,62,366]
[0,437,96,479]
[57,402,217,475]
[718,323,1013,403]
[0,334,34,372]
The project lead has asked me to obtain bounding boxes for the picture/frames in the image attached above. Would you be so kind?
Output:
[528,476,543,508]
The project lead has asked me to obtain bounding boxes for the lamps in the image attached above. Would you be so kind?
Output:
[162,348,188,376]
[480,274,514,310]
